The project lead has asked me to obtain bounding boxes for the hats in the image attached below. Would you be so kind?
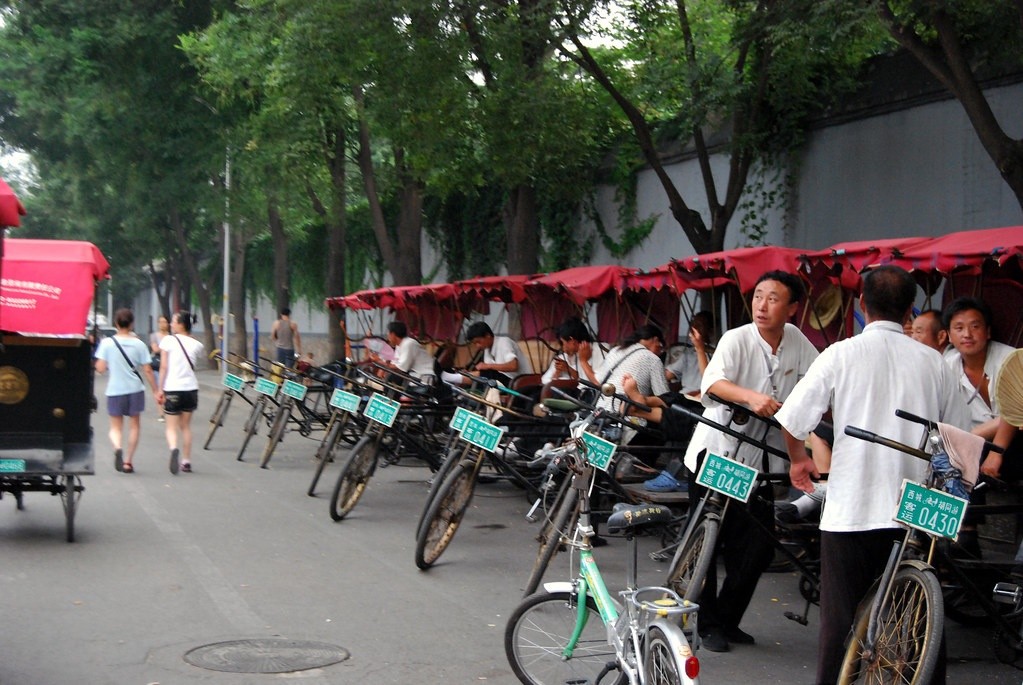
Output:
[810,282,842,329]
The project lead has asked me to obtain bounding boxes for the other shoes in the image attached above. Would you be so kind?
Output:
[702,629,729,652]
[433,359,443,379]
[591,536,607,547]
[775,503,804,524]
[727,627,754,645]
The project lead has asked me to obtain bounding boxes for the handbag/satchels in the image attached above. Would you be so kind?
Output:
[151,354,160,372]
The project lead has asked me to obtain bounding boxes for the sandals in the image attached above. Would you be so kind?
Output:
[645,471,689,492]
[121,463,134,474]
[115,448,124,472]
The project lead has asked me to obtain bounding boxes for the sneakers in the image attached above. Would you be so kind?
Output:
[170,448,180,475]
[180,464,191,472]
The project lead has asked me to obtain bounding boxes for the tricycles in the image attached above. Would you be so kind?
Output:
[504,409,710,685]
[0,234,111,532]
[667,233,933,631]
[517,245,855,622]
[208,247,688,574]
[837,225,1022,685]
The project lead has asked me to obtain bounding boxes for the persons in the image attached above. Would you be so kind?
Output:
[432,322,533,395]
[370,321,435,401]
[621,312,713,428]
[533,319,612,416]
[594,325,671,428]
[683,270,831,652]
[910,297,1023,559]
[94,309,158,473]
[303,353,314,385]
[150,309,203,474]
[774,265,974,685]
[272,308,300,368]
